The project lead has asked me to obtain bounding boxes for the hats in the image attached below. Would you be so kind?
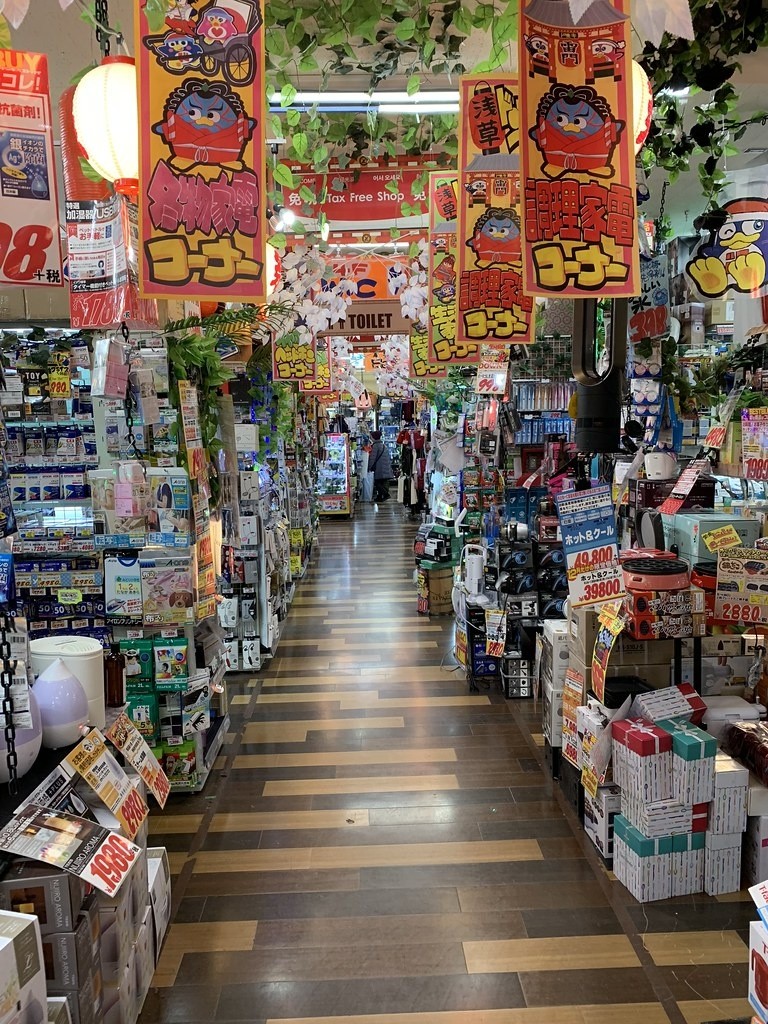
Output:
[371,431,383,440]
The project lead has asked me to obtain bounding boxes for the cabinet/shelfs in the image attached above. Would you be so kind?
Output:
[317,433,351,516]
[226,387,283,674]
[0,330,230,795]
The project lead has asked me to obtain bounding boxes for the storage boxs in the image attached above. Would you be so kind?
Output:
[745,814,768,885]
[89,775,172,1024]
[558,751,584,822]
[654,717,718,807]
[47,951,104,1024]
[584,786,621,872]
[0,861,86,935]
[611,717,674,804]
[704,831,742,897]
[747,775,768,816]
[708,746,749,834]
[544,738,562,782]
[0,909,48,1024]
[41,893,102,991]
[0,236,768,748]
[613,815,672,903]
[620,788,708,840]
[747,921,768,1024]
[47,997,72,1024]
[671,832,704,898]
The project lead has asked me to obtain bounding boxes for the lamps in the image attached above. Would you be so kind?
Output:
[266,209,284,232]
[273,204,294,223]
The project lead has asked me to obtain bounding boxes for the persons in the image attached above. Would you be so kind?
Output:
[155,477,172,508]
[369,432,392,501]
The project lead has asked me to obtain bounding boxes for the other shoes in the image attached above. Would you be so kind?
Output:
[374,495,383,501]
[366,402,369,406]
[382,493,391,503]
[360,403,362,406]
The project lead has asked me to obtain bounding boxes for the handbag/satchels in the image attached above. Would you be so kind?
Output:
[371,463,376,472]
